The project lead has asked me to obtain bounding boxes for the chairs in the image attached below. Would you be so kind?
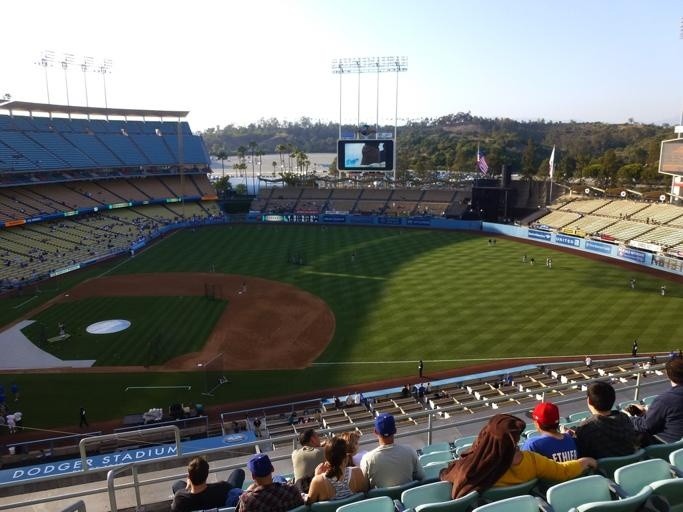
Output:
[250,189,472,216]
[241,393,683,512]
[1,115,221,280]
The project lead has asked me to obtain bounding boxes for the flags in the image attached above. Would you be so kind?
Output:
[477,145,488,173]
[548,147,554,178]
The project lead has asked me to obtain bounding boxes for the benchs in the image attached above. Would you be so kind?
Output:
[265,360,666,451]
[539,193,683,251]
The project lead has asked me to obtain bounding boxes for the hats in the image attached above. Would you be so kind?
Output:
[375,413,395,436]
[526,402,559,426]
[248,454,271,476]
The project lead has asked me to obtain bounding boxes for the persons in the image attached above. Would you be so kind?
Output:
[171,412,426,512]
[631,340,638,357]
[8,444,47,462]
[333,392,365,411]
[0,145,220,288]
[629,276,639,291]
[493,371,513,389]
[230,407,322,438]
[520,403,577,462]
[660,282,667,297]
[481,235,558,269]
[624,357,683,447]
[419,360,424,378]
[668,350,676,361]
[1,381,24,434]
[79,407,89,427]
[572,383,636,460]
[221,179,541,224]
[402,382,432,397]
[633,352,657,370]
[182,401,203,418]
[440,413,597,488]
[585,355,592,368]
